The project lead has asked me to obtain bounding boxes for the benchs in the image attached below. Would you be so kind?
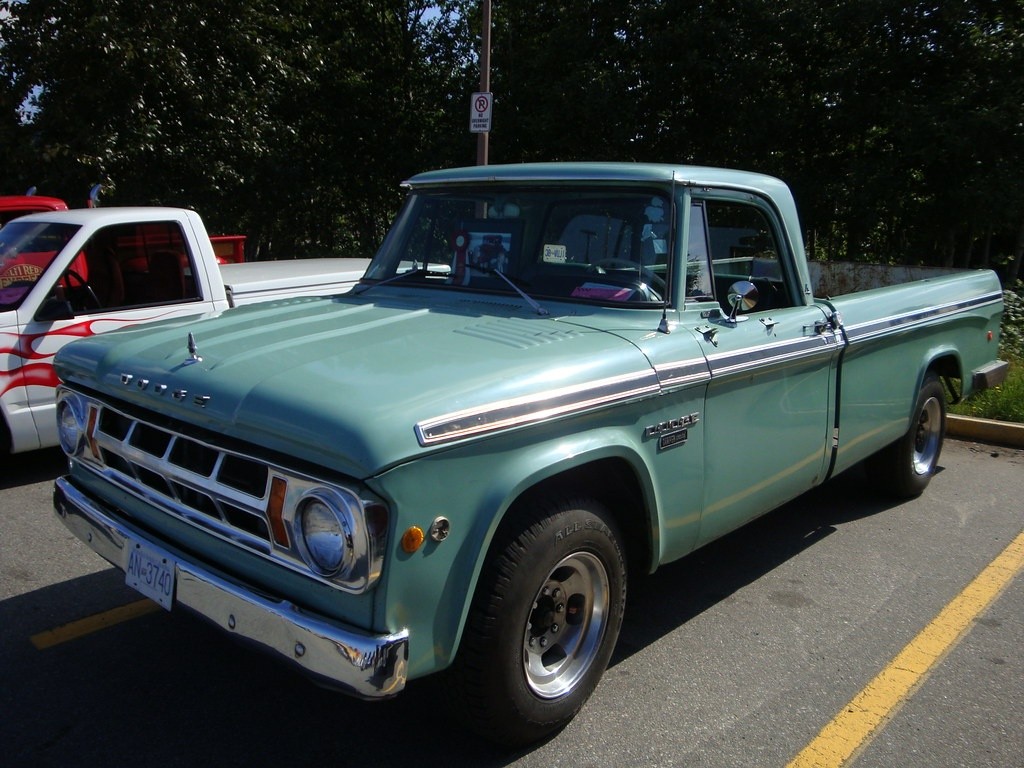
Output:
[537,261,787,318]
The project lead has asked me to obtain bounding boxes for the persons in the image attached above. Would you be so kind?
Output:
[489,252,508,276]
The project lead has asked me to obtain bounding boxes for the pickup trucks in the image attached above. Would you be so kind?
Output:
[0,202,452,486]
[0,195,248,315]
[53,160,1010,756]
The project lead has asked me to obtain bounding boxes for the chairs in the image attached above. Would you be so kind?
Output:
[146,250,186,302]
[86,249,126,308]
